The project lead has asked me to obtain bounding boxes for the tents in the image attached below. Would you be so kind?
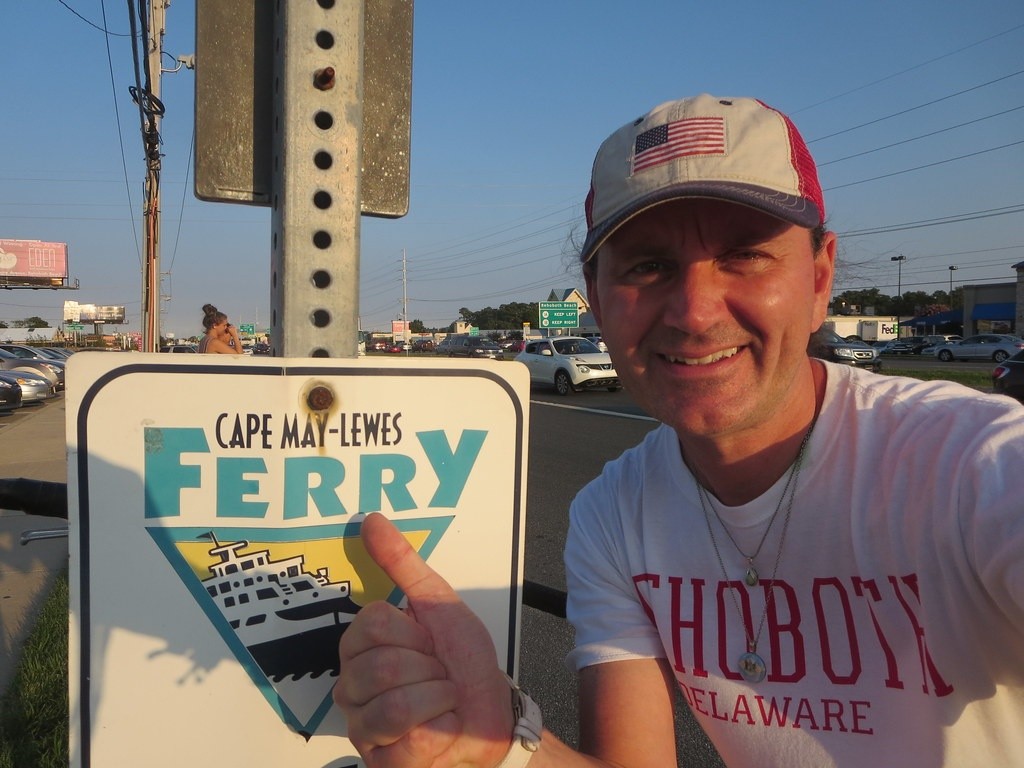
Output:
[899,312,962,336]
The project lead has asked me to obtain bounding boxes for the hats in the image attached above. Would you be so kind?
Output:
[579,91,826,264]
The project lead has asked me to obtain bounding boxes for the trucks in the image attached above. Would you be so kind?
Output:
[357,330,372,356]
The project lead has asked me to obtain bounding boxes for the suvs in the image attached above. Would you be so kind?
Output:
[448,335,505,361]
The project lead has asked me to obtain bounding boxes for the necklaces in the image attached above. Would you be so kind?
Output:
[695,419,814,683]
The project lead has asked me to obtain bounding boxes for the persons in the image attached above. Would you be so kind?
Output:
[339,90,1024,768]
[199,303,244,354]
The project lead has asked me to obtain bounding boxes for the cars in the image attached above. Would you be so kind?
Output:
[365,339,456,355]
[806,328,883,374]
[934,334,1024,363]
[1,340,78,417]
[499,339,527,353]
[872,335,965,355]
[992,348,1024,407]
[161,342,269,356]
[513,335,621,397]
[580,337,608,352]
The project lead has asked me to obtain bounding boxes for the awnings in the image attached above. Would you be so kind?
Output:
[970,303,1015,320]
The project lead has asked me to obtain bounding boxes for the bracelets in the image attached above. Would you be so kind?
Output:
[495,668,542,768]
